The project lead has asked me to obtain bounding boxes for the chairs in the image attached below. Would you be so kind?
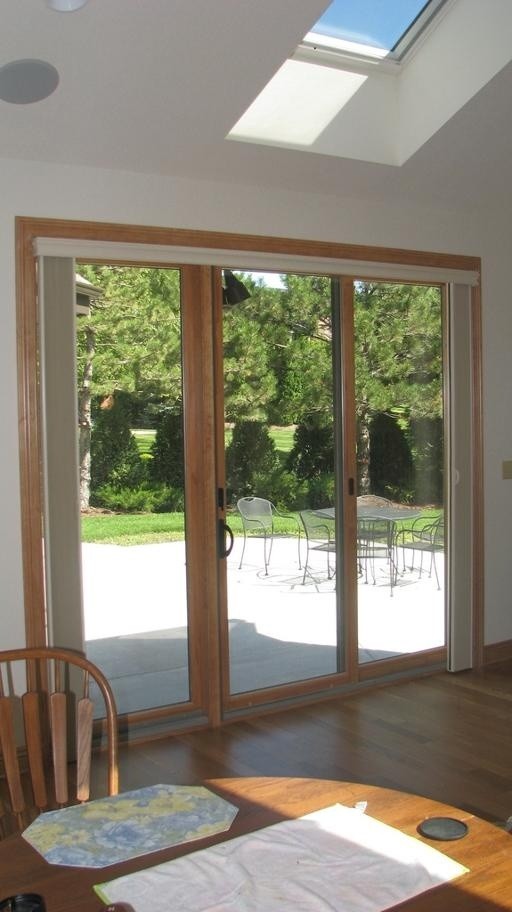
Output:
[236,497,445,598]
[1,648,119,830]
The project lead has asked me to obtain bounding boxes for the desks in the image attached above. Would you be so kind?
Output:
[0,777,512,911]
